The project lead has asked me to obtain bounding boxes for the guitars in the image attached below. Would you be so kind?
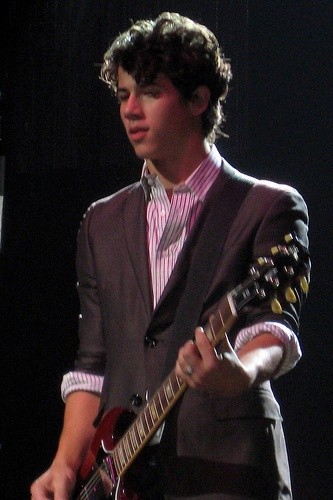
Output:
[67,219,313,500]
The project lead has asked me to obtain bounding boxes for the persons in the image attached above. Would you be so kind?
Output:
[31,11,312,500]
